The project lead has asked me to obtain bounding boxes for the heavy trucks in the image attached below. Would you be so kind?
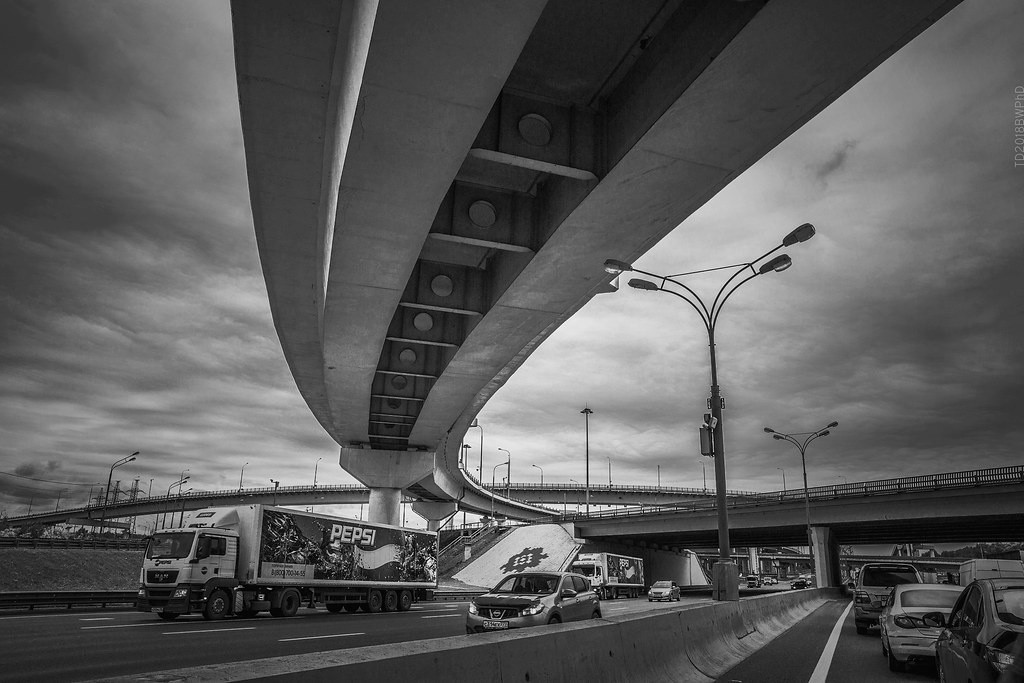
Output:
[136,503,440,621]
[569,552,645,600]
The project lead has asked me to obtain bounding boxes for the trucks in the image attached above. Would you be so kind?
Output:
[960,559,1024,588]
[746,575,761,588]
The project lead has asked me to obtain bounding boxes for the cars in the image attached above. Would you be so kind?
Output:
[647,580,681,603]
[772,579,778,584]
[870,583,964,671]
[789,575,814,590]
[921,578,1024,683]
[466,571,602,634]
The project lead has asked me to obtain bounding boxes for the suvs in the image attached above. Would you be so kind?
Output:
[847,563,924,634]
[764,577,772,586]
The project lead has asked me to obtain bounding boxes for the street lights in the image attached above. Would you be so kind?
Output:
[463,444,473,472]
[604,221,816,601]
[763,421,840,590]
[498,447,512,484]
[533,464,543,488]
[162,476,191,531]
[492,462,510,526]
[270,478,280,506]
[579,402,594,519]
[179,469,190,496]
[699,461,706,493]
[149,478,154,499]
[99,451,140,533]
[313,457,323,487]
[777,467,786,492]
[238,463,250,494]
[606,456,612,491]
